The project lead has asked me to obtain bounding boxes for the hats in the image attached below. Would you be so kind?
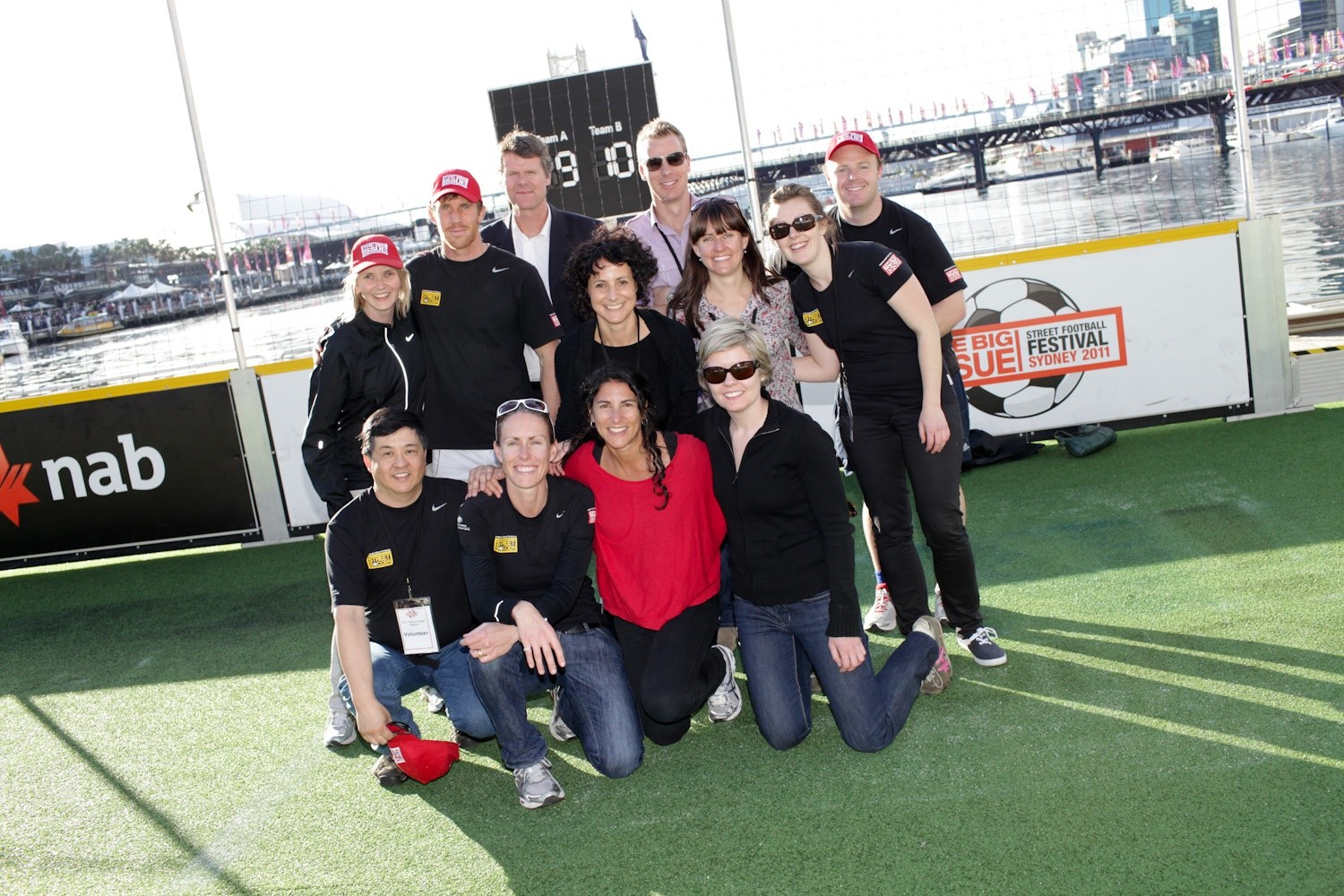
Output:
[826,131,880,162]
[351,235,403,274]
[431,169,482,203]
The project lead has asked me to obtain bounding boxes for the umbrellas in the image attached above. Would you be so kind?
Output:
[6,301,55,313]
[101,280,186,309]
[324,262,350,277]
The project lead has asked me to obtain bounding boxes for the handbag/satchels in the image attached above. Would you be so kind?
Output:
[1055,423,1115,457]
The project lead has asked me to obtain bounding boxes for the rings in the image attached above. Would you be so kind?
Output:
[524,648,530,652]
[477,649,482,656]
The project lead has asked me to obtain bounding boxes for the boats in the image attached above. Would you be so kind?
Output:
[1149,113,1344,163]
[57,313,124,337]
[0,322,29,358]
[916,143,1110,195]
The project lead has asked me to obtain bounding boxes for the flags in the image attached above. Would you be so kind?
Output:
[205,235,311,274]
[756,28,1344,146]
[630,10,649,61]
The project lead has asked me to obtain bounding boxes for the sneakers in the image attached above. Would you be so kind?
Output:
[324,712,357,747]
[423,686,443,712]
[707,645,741,722]
[913,615,952,695]
[375,755,408,784]
[957,625,1005,666]
[549,686,576,741]
[934,595,948,623]
[864,583,897,631]
[513,756,564,810]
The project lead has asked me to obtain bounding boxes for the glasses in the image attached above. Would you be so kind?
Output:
[690,195,741,217]
[703,360,761,384]
[495,398,555,442]
[640,151,687,171]
[770,214,825,239]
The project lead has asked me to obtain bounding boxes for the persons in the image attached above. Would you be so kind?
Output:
[825,130,969,633]
[554,226,699,442]
[301,234,446,748]
[666,195,811,652]
[760,183,1007,667]
[312,168,565,482]
[479,131,604,401]
[16,301,132,332]
[324,409,497,787]
[616,118,697,314]
[484,361,742,747]
[460,398,645,809]
[549,317,953,752]
